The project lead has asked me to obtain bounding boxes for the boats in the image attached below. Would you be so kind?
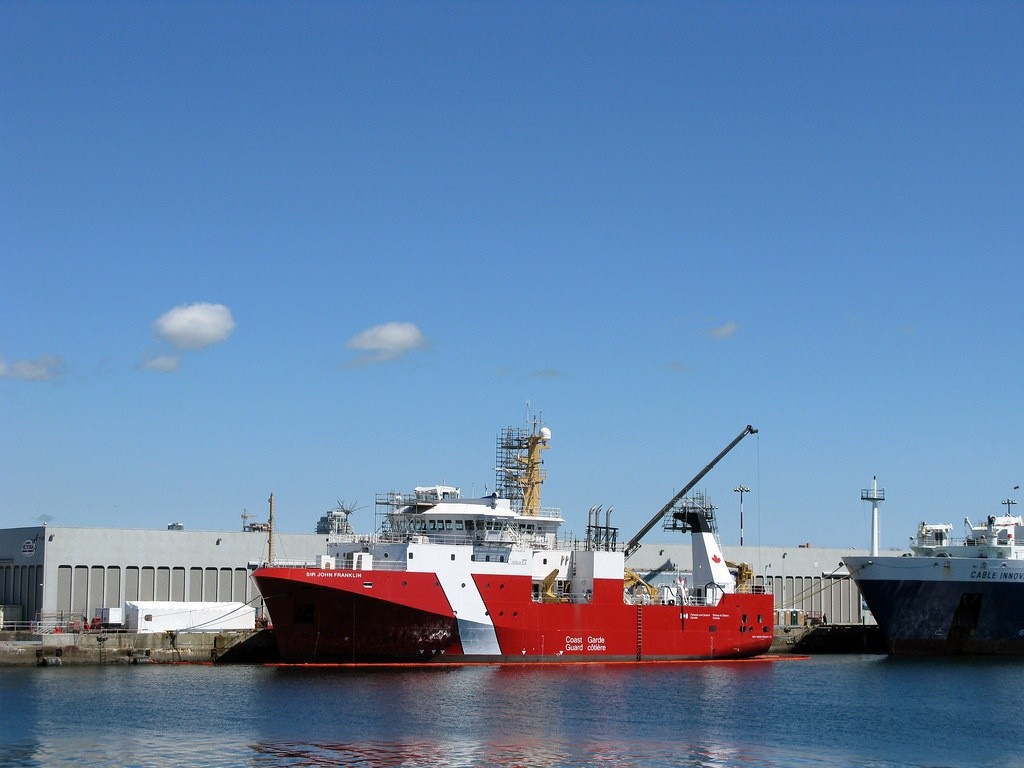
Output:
[841,474,1023,655]
[217,400,774,667]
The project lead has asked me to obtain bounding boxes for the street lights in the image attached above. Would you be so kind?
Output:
[733,484,751,546]
